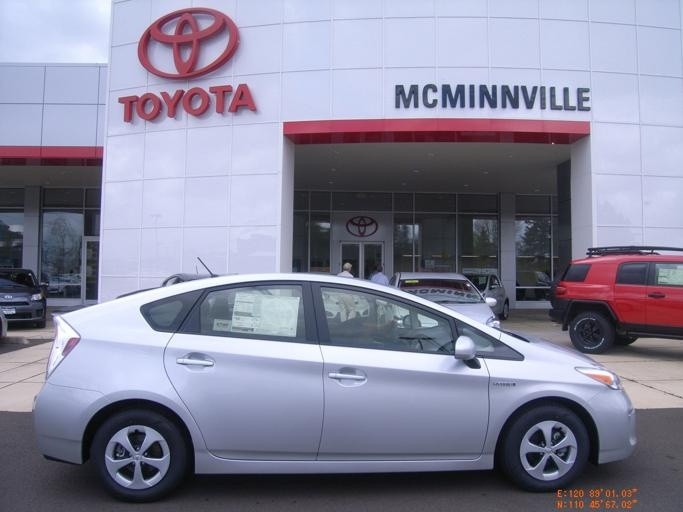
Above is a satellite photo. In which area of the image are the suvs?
[548,243,682,355]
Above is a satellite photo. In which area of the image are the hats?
[343,263,352,269]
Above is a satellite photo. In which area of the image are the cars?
[374,268,501,334]
[31,258,638,503]
[0,268,48,329]
[462,269,510,320]
[516,271,550,300]
[42,276,81,297]
[0,309,7,339]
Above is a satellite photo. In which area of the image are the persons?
[338,262,353,278]
[371,266,389,287]
